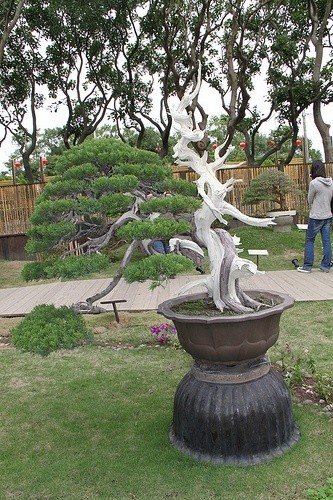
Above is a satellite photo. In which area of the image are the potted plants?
[241,169,304,233]
[158,288,302,468]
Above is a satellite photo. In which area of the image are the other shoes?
[297,266,311,272]
[318,268,328,272]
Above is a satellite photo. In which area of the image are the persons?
[155,240,172,283]
[297,160,332,273]
